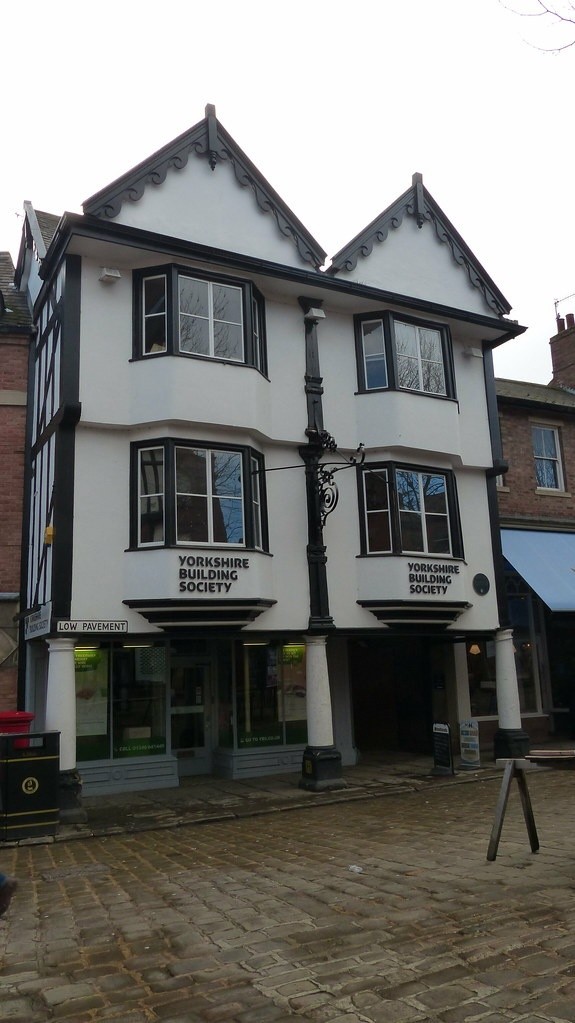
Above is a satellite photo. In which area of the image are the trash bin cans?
[0,729,62,841]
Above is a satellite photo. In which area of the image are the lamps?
[464,347,484,358]
[304,308,326,324]
[100,267,121,283]
